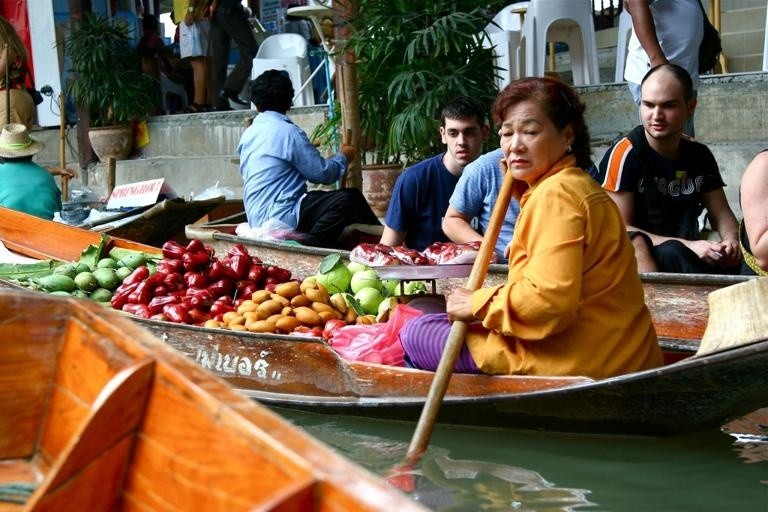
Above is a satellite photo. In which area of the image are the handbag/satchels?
[697,1,722,75]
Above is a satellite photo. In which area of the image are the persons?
[235,68,382,248]
[736,149,768,278]
[0,122,65,222]
[396,76,666,382]
[42,164,81,181]
[596,63,742,274]
[439,144,525,263]
[620,0,706,139]
[0,20,36,138]
[130,1,338,113]
[377,97,490,253]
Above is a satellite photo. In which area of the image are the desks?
[286,3,339,107]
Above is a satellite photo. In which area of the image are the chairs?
[160,70,188,115]
[464,0,601,89]
[248,34,315,112]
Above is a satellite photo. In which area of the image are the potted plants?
[315,0,510,221]
[58,9,157,162]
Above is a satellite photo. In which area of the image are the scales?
[373,264,474,370]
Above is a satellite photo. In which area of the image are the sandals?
[184,102,215,113]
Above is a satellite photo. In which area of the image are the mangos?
[39,254,147,301]
[205,283,376,332]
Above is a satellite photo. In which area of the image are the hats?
[0,123,45,158]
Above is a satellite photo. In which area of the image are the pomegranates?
[303,261,427,314]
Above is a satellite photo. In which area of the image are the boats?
[0,194,224,253]
[1,207,767,435]
[185,210,767,351]
[0,290,430,510]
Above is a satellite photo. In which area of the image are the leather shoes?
[219,88,248,105]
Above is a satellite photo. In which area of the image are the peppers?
[111,240,292,327]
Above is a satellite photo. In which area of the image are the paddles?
[383,169,515,495]
